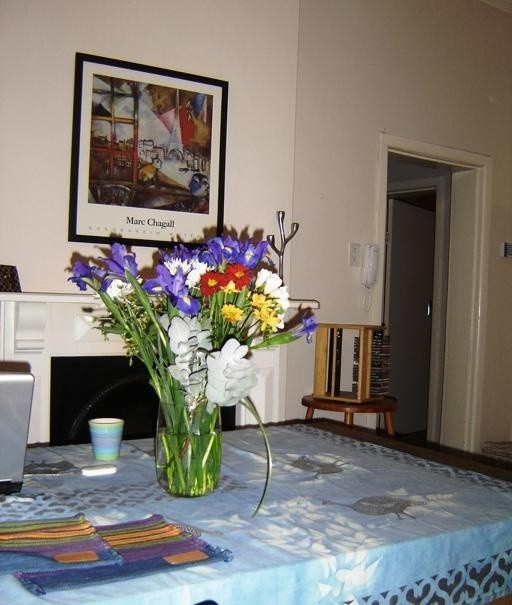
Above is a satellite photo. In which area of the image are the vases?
[156,398,221,497]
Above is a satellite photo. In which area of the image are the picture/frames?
[67,51,229,255]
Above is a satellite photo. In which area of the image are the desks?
[2,416,511,605]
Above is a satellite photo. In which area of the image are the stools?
[302,394,397,438]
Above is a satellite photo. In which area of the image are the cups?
[88,417,126,463]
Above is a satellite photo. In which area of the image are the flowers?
[63,237,319,519]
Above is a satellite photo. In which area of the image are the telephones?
[361,243,378,288]
